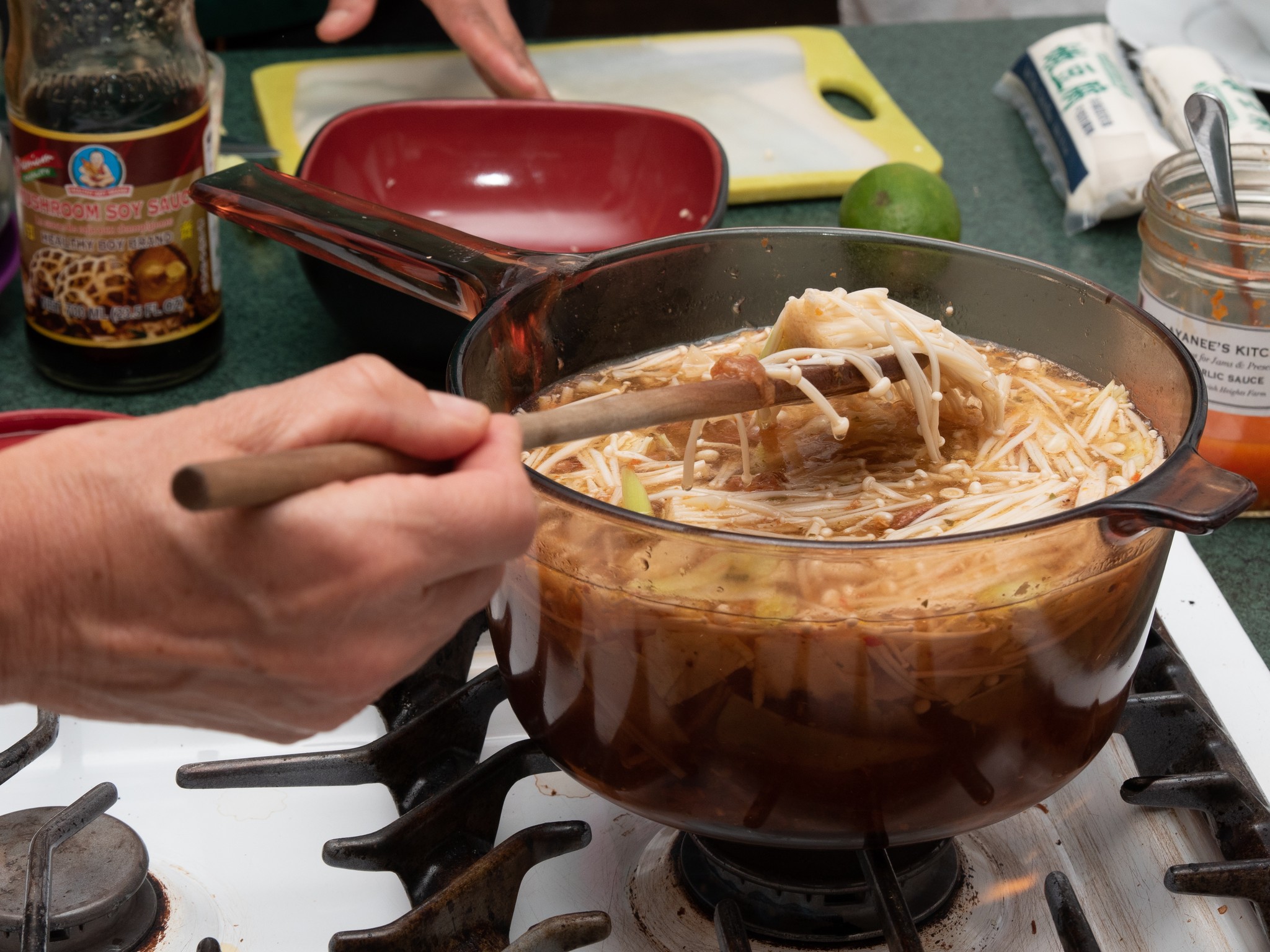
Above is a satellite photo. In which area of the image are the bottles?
[2,0,226,394]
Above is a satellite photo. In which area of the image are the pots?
[188,161,1261,849]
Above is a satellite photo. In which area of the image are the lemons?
[837,162,958,290]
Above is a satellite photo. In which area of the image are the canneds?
[1137,141,1269,521]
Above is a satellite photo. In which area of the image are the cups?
[65,41,226,178]
[1137,143,1270,518]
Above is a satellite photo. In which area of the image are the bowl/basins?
[296,98,730,396]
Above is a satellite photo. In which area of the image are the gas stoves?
[0,524,1270,952]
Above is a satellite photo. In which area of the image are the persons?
[0,0,556,745]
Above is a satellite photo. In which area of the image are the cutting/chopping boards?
[250,26,944,205]
